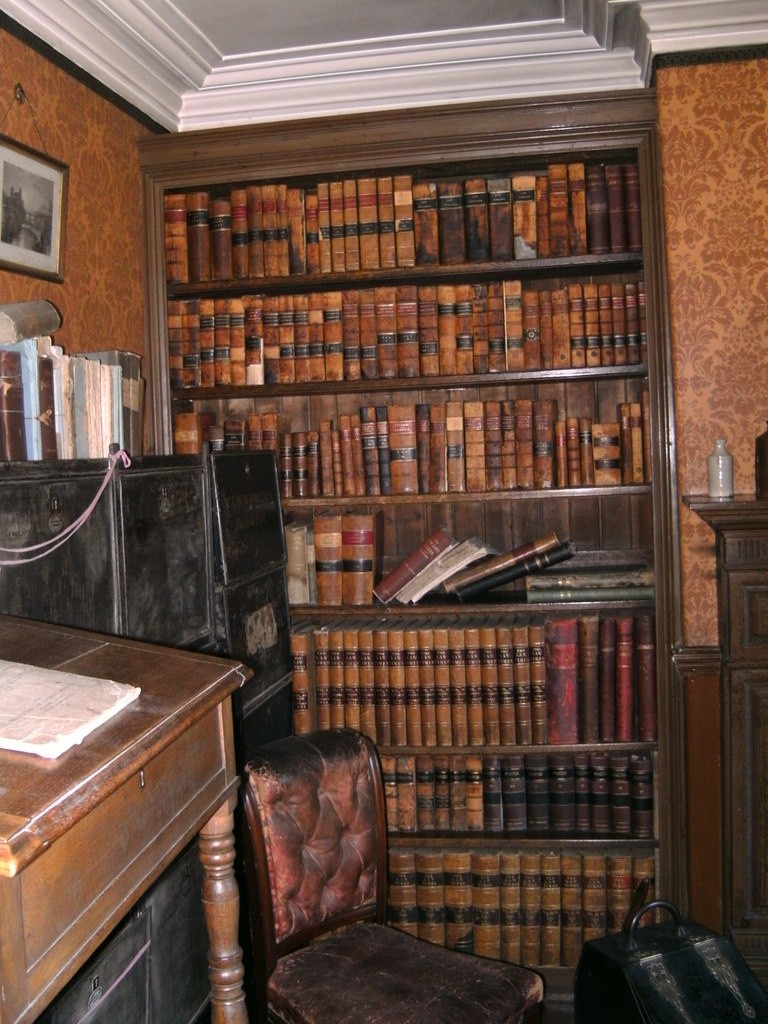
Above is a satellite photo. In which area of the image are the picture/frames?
[0,133,70,284]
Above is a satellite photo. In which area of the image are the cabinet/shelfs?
[133,86,681,1016]
[0,442,294,1024]
[682,493,768,989]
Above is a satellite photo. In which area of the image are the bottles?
[708,438,733,497]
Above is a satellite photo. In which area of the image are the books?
[173,390,651,502]
[387,843,656,970]
[292,611,656,747]
[161,162,653,285]
[167,280,649,389]
[1,334,147,461]
[378,751,654,838]
[285,510,656,605]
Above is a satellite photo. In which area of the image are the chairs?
[232,727,545,1024]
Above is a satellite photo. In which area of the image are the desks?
[0,613,249,1024]
